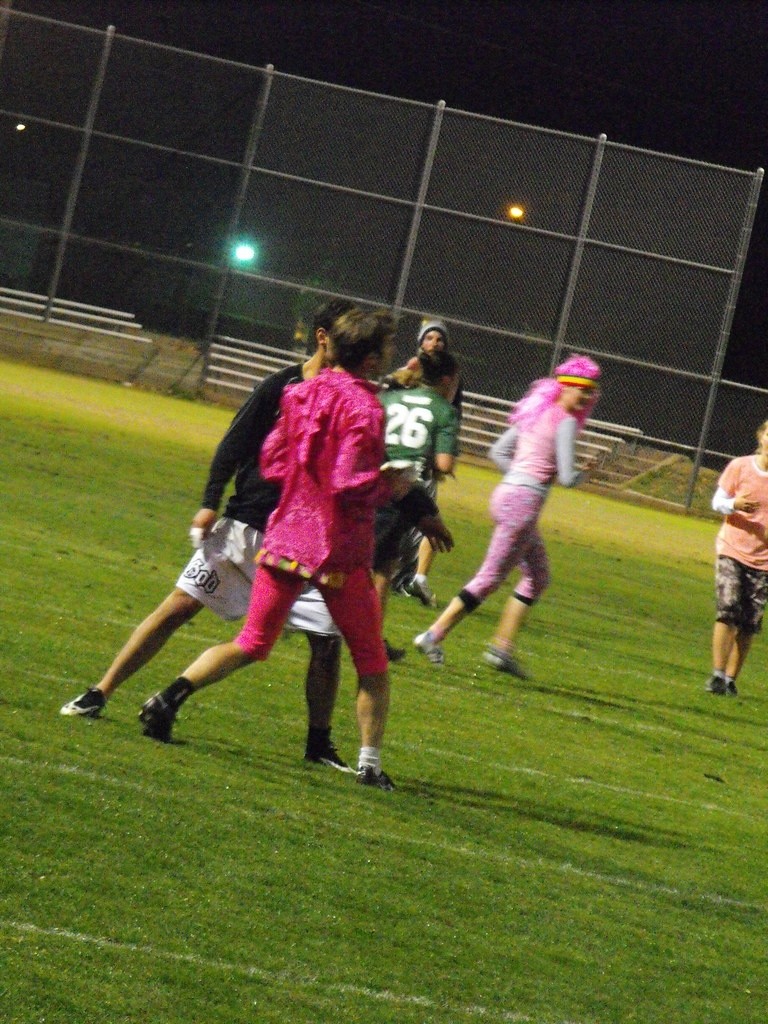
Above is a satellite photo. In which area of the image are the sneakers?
[355,766,394,790]
[60,690,104,718]
[482,647,532,681]
[304,749,355,773]
[414,632,447,665]
[138,694,172,743]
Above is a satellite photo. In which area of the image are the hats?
[417,321,450,350]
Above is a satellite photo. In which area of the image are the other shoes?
[725,678,736,696]
[405,581,437,608]
[703,676,724,694]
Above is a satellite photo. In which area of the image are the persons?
[704,420,768,698]
[138,304,440,792]
[59,301,456,774]
[369,318,603,681]
